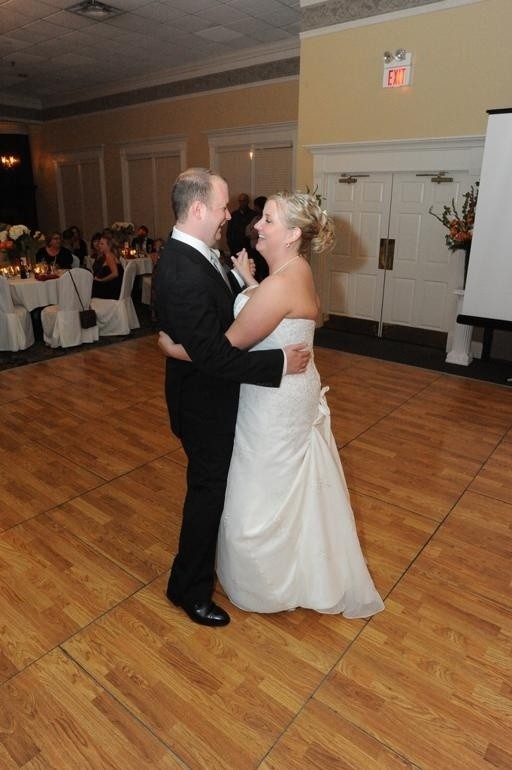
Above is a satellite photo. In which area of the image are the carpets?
[0,298,162,370]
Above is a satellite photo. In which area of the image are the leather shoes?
[165,589,230,627]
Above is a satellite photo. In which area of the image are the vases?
[445,289,473,367]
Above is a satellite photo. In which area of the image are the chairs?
[0,253,152,352]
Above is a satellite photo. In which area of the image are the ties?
[209,248,233,296]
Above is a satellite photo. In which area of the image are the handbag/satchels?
[80,309,97,329]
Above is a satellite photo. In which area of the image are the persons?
[245,196,267,283]
[152,165,312,627]
[35,225,165,305]
[226,193,257,257]
[157,188,384,620]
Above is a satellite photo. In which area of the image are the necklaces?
[272,255,299,274]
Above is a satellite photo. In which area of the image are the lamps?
[82,4,109,18]
[384,49,412,68]
[1,152,17,169]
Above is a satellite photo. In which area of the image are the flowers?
[111,222,135,246]
[429,181,479,290]
[0,223,47,264]
